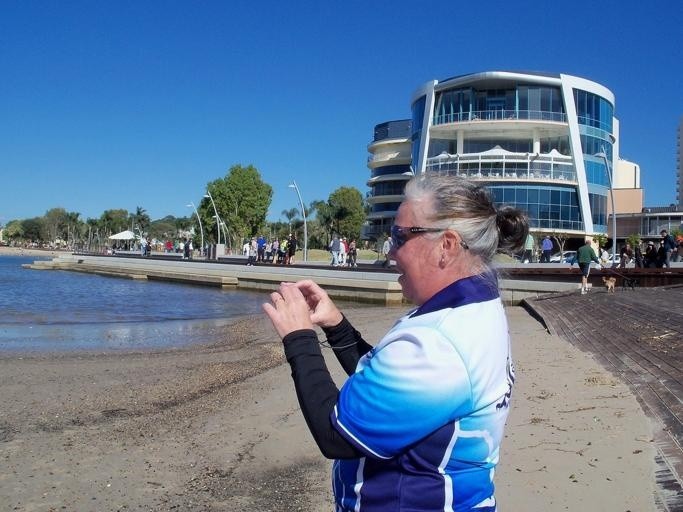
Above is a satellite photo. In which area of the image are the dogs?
[602,277,617,294]
[621,278,636,292]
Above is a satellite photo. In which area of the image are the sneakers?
[585,287,591,291]
[581,291,587,295]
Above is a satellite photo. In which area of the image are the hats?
[343,237,347,239]
[648,241,654,246]
[339,238,343,242]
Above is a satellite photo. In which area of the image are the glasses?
[390,225,469,249]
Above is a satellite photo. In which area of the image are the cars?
[529,249,621,266]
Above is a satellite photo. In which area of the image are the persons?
[600,246,609,263]
[164,237,193,260]
[539,236,553,263]
[112,236,152,256]
[569,240,600,294]
[620,243,632,268]
[635,230,679,268]
[382,235,394,268]
[521,232,534,263]
[262,171,528,512]
[329,234,358,268]
[310,232,327,249]
[243,232,296,266]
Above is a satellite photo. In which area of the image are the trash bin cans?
[208,244,225,260]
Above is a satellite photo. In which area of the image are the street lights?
[593,144,616,266]
[186,201,203,257]
[134,227,142,238]
[203,190,231,250]
[287,178,307,262]
